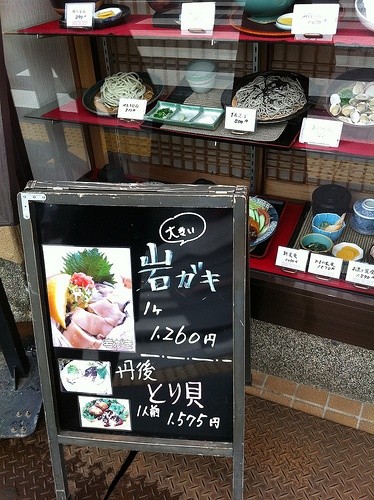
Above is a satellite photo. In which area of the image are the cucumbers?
[248,208,267,233]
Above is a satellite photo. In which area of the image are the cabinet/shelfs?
[0,0,374,349]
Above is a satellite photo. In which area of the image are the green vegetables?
[339,89,355,106]
[304,242,327,252]
[249,200,263,209]
[67,363,108,379]
[62,247,118,286]
[154,108,174,119]
[82,399,128,422]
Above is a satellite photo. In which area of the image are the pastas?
[235,76,307,121]
[99,71,147,108]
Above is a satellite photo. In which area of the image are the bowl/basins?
[49,0,104,18]
[234,0,294,23]
[145,0,195,14]
[325,68,374,136]
[354,0,374,32]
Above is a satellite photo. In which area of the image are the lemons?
[48,274,73,329]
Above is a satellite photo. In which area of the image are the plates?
[249,196,279,247]
[58,4,131,28]
[60,360,109,395]
[93,7,121,19]
[82,72,164,117]
[220,70,317,124]
[229,0,346,37]
[143,100,225,130]
[332,241,364,265]
[152,0,237,28]
[349,215,374,235]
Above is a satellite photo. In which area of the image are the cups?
[183,59,217,94]
[311,213,346,242]
[353,199,374,231]
[312,184,351,219]
[300,233,334,260]
[97,164,123,183]
